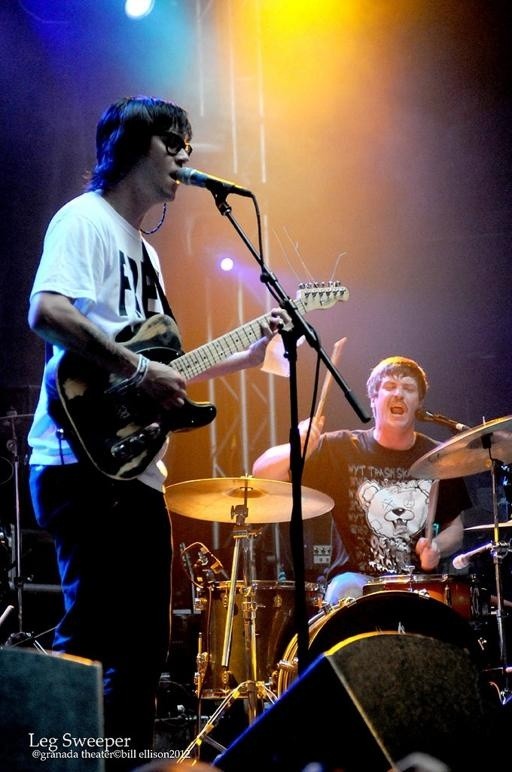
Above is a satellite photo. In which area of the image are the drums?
[200,578,326,700]
[364,571,492,640]
[274,592,480,701]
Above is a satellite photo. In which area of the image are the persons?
[22,95,290,770]
[252,355,512,612]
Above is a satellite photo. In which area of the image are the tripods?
[0,411,48,655]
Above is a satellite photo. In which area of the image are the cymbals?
[164,478,334,526]
[1,414,35,427]
[410,413,512,481]
[464,517,512,530]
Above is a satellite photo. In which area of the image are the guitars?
[44,280,350,482]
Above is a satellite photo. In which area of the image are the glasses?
[153,130,193,157]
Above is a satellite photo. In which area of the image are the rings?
[276,320,286,333]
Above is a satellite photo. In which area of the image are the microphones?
[452,543,492,569]
[414,408,470,432]
[175,167,251,198]
